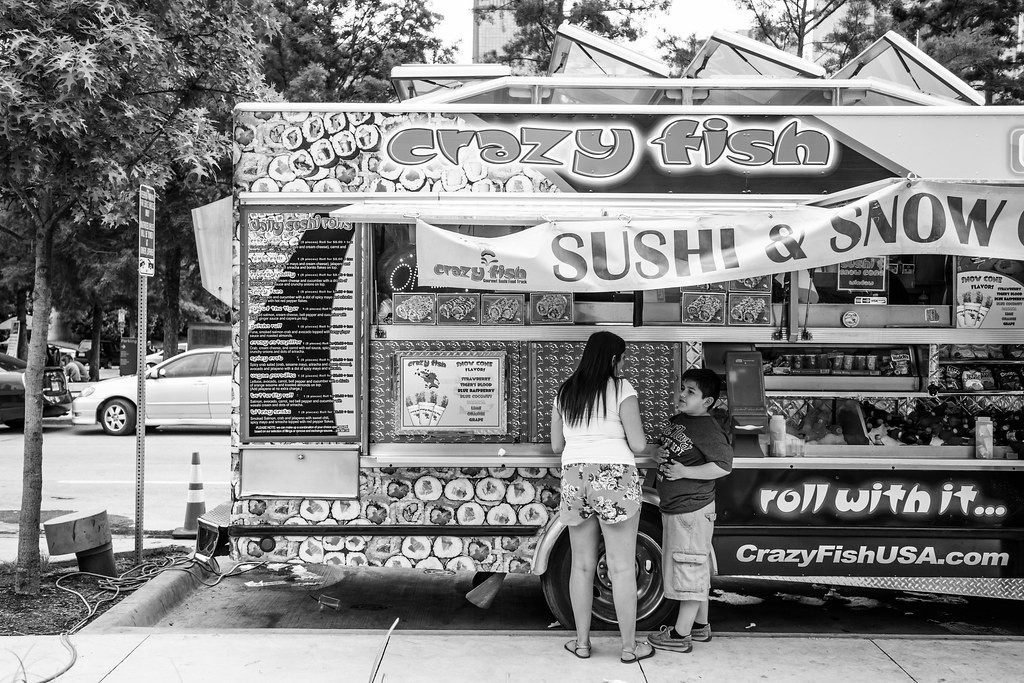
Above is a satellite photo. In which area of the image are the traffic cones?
[172,451,206,540]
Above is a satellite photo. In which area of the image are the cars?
[70,346,233,435]
[0,353,72,429]
[146,345,186,370]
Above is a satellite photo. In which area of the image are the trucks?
[190,23,1024,632]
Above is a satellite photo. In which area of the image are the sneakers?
[691,623,712,642]
[646,625,693,653]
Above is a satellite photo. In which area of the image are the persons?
[645,368,733,654]
[550,330,656,664]
[60,355,92,380]
[772,270,818,304]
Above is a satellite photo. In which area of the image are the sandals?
[565,640,591,658]
[621,641,655,664]
